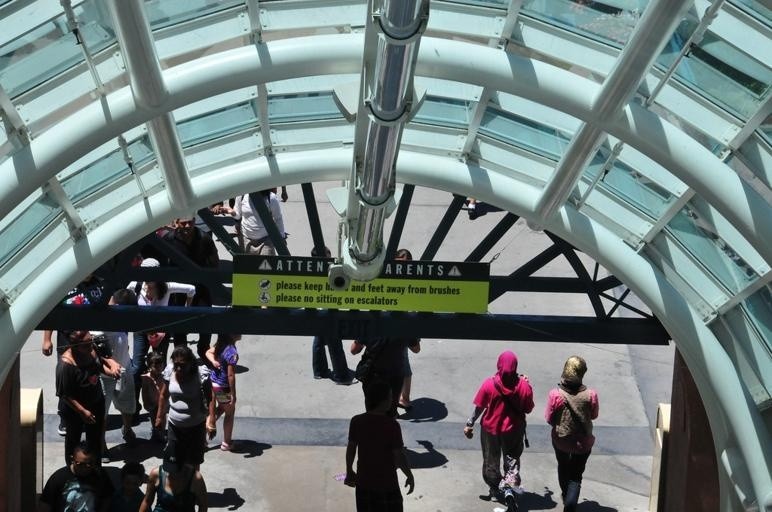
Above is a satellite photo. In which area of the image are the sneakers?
[504,489,518,512]
[490,488,500,502]
[221,439,242,451]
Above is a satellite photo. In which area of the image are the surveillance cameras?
[327,265,352,289]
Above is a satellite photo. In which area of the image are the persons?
[466,198,477,216]
[210,186,292,256]
[309,244,359,387]
[344,377,417,512]
[395,249,413,263]
[350,334,418,420]
[462,350,533,510]
[38,217,241,512]
[544,354,599,510]
